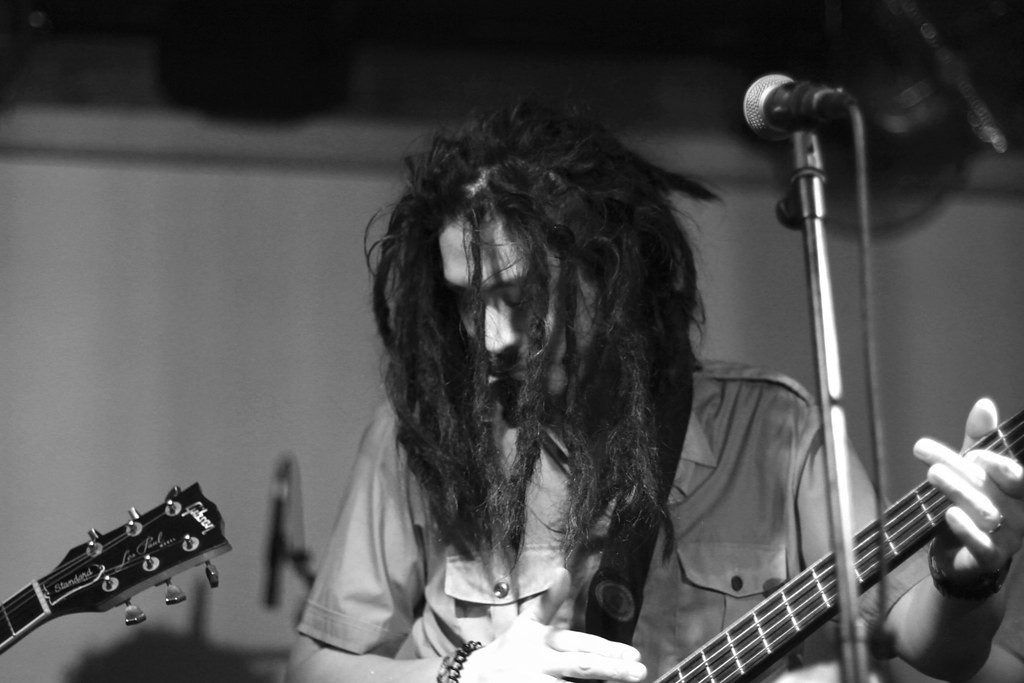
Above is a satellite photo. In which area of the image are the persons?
[285,93,1024,683]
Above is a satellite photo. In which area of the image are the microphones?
[744,73,854,142]
[261,472,289,615]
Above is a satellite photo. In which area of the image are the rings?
[988,516,1005,534]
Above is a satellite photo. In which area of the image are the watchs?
[435,640,482,683]
[928,533,1012,601]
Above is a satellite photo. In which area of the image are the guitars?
[0,479,234,660]
[656,412,1022,683]
